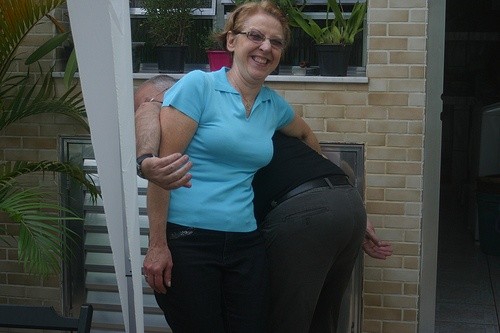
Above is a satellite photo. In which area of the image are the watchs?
[136,153,160,179]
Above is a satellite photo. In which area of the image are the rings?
[144,274,148,278]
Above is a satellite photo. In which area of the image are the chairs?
[465,102,500,246]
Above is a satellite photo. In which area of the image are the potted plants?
[141,0,195,74]
[198,27,232,71]
[60,13,78,72]
[294,0,366,77]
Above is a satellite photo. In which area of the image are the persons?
[134,74,394,333]
[144,0,322,333]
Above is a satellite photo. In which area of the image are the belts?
[257,177,350,219]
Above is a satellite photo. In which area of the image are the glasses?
[144,85,172,102]
[235,30,287,49]
